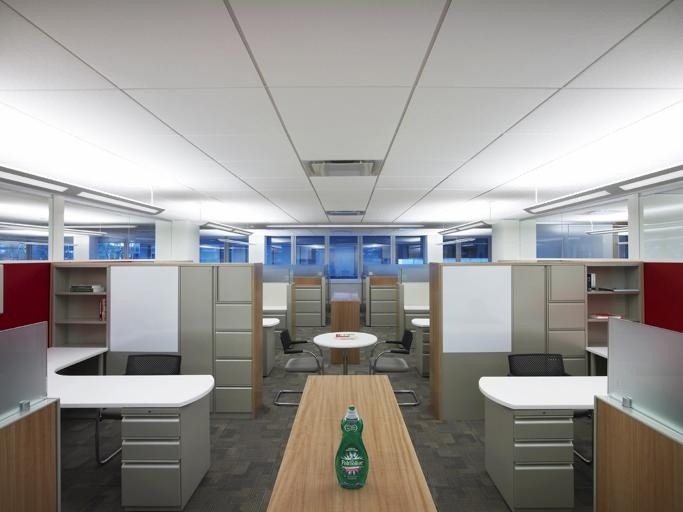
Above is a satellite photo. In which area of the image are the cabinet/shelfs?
[586,259,644,346]
[52,262,110,349]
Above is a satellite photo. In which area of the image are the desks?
[313,332,377,375]
[330,292,362,332]
[263,306,286,378]
[47,347,215,512]
[404,304,429,377]
[479,347,607,512]
[266,376,436,512]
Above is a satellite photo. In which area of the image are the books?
[70,284,100,288]
[69,287,104,292]
[101,298,106,321]
[598,287,640,292]
[99,301,101,320]
[591,273,595,291]
[335,333,355,339]
[586,273,591,291]
[592,312,623,320]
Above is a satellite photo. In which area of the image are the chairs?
[97,355,180,467]
[508,354,593,463]
[273,330,324,406]
[369,328,420,406]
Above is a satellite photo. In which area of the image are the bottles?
[334,403,369,490]
